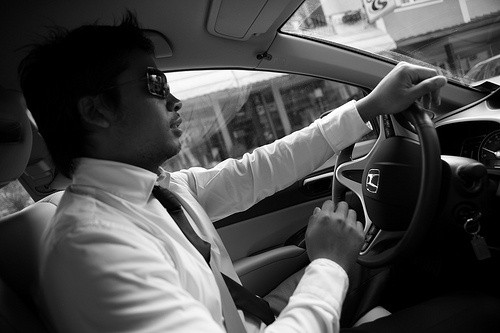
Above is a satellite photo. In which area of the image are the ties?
[152,184,276,326]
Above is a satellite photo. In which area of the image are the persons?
[19,10,446,333]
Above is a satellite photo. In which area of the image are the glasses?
[90,67,169,99]
